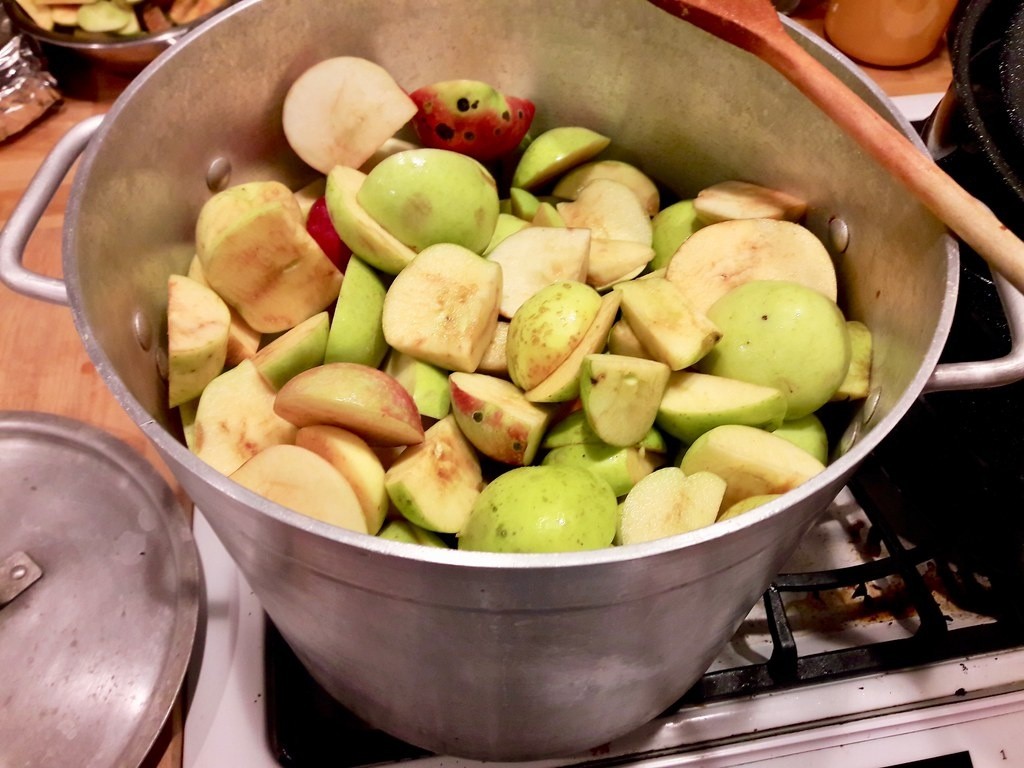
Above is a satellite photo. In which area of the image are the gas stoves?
[181,83,1022,768]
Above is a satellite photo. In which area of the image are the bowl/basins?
[9,0,236,74]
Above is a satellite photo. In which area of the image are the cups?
[824,0,954,68]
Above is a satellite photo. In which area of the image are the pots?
[3,0,1022,761]
